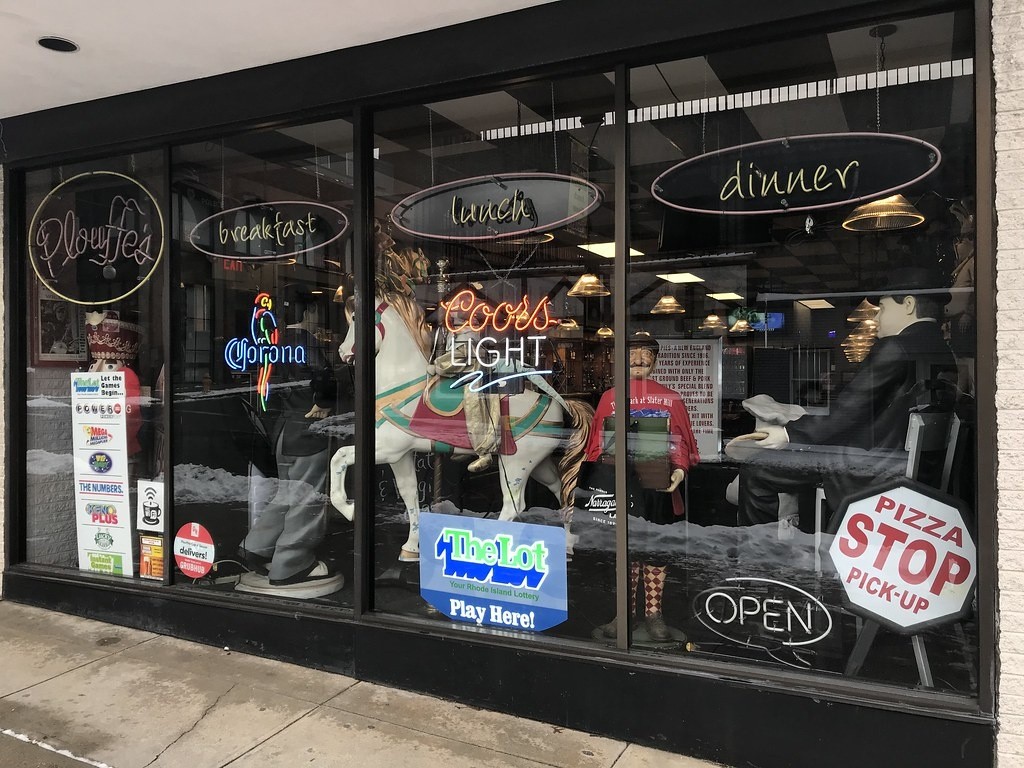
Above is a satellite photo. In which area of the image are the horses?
[329,272,596,564]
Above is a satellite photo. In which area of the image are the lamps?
[729,264,755,333]
[505,281,531,324]
[333,283,344,304]
[840,337,874,347]
[593,321,614,339]
[698,268,727,328]
[566,150,611,296]
[841,25,925,232]
[649,273,686,314]
[849,320,881,336]
[844,346,870,363]
[846,298,880,322]
[556,291,581,331]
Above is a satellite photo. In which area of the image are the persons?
[85,319,145,572]
[584,334,700,643]
[738,267,958,535]
[236,284,337,586]
